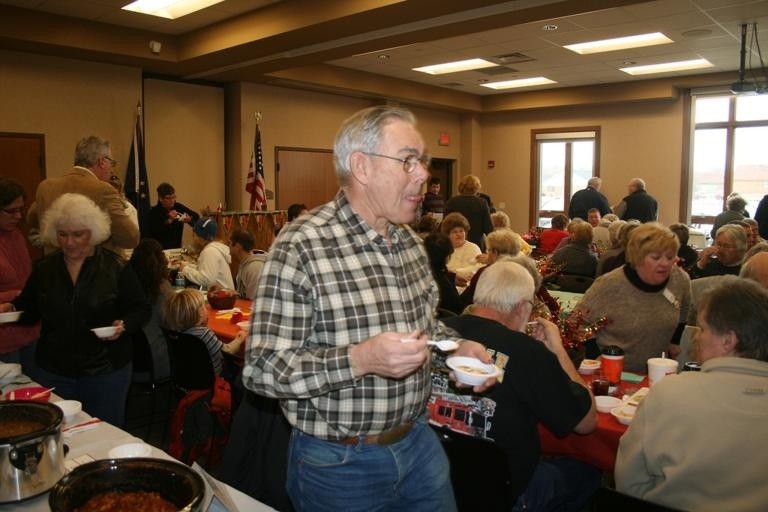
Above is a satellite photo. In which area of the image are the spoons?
[402,336,458,354]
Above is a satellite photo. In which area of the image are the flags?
[126,109,149,209]
[246,123,271,212]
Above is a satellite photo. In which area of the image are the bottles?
[591,378,609,396]
[175,270,185,289]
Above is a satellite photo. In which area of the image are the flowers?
[521,224,543,247]
[532,257,562,278]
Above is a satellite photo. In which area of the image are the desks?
[158,287,654,472]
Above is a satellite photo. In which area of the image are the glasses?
[163,195,178,200]
[485,248,494,255]
[106,156,117,167]
[1,206,29,214]
[528,300,537,315]
[361,150,432,174]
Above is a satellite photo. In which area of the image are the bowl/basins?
[593,396,623,415]
[235,320,251,333]
[206,290,237,311]
[446,354,500,387]
[48,457,205,511]
[0,311,24,323]
[89,326,119,337]
[0,363,83,418]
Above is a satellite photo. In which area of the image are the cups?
[599,344,625,393]
[682,361,702,372]
[645,358,678,390]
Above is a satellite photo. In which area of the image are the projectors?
[730,82,764,94]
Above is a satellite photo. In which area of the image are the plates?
[614,387,650,424]
[576,359,601,375]
[107,443,151,459]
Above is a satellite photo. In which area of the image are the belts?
[341,421,415,447]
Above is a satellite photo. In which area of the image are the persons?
[1,194,151,430]
[444,175,495,241]
[242,102,509,512]
[152,183,200,249]
[569,177,613,222]
[125,192,768,512]
[610,178,658,223]
[612,274,767,511]
[480,191,496,215]
[422,178,445,213]
[25,136,141,262]
[0,179,40,370]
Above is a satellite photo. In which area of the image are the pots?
[0,398,70,504]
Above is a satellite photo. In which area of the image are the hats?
[193,216,217,242]
